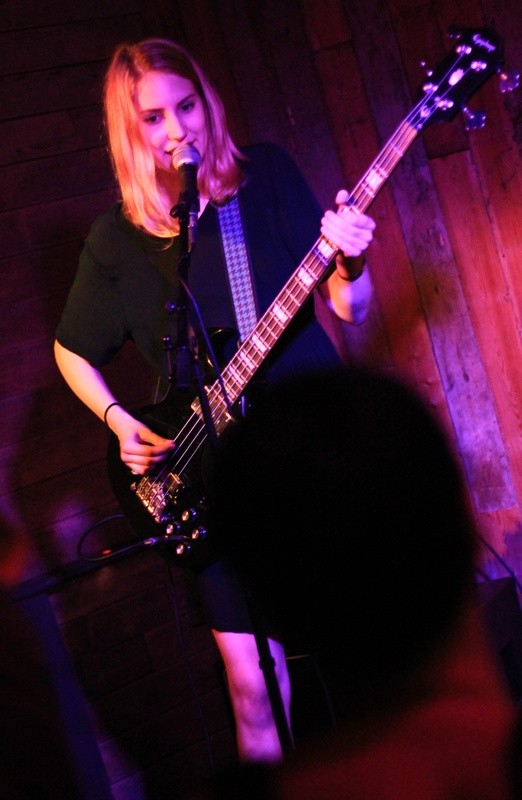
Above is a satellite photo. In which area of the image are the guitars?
[105,22,522,568]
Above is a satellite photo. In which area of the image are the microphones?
[172,144,204,244]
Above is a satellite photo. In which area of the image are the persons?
[54,40,378,759]
[195,364,521,800]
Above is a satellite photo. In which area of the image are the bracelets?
[337,256,367,282]
[104,402,122,427]
